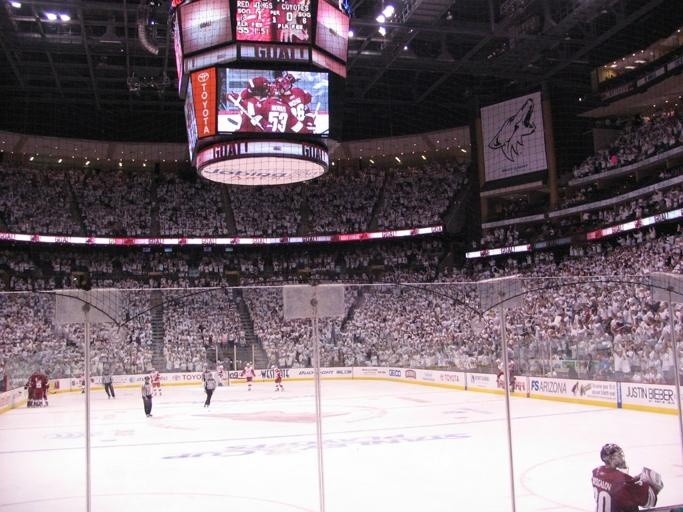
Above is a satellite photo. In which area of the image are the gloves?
[249,114,266,131]
[304,116,316,131]
[224,91,240,103]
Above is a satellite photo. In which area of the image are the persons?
[592,442,658,512]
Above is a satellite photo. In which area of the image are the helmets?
[274,78,290,93]
[246,76,270,97]
[600,443,621,464]
